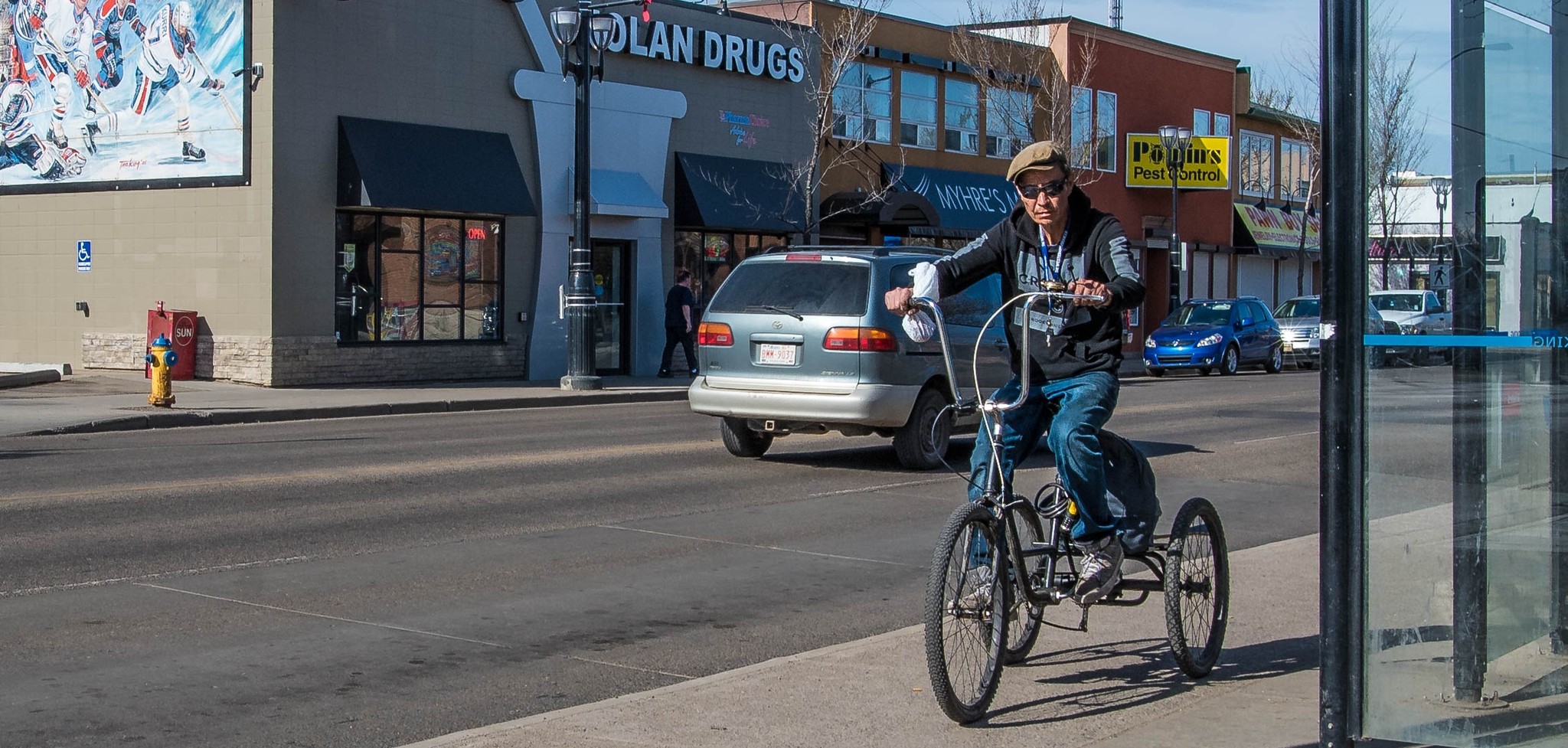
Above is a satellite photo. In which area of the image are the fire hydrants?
[144,332,178,408]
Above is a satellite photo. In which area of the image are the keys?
[1045,328,1054,347]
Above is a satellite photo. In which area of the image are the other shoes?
[689,369,699,378]
[658,370,674,378]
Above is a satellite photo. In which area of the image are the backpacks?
[1098,429,1162,553]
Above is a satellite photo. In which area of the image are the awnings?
[1233,203,1320,262]
[674,152,819,233]
[880,163,1021,238]
[337,117,539,216]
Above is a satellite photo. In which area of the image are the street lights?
[1157,124,1194,315]
[1430,176,1452,314]
[547,0,617,392]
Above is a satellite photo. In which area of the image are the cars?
[1140,295,1284,377]
[1271,294,1389,372]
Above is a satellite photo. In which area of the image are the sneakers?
[1074,535,1124,603]
[947,567,1018,620]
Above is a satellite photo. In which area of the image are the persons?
[885,141,1145,620]
[657,271,699,378]
[592,275,606,332]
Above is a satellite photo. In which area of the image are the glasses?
[1016,176,1068,199]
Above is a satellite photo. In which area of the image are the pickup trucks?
[1369,289,1453,368]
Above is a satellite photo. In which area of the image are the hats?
[1006,141,1063,185]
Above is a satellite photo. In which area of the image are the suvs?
[687,241,1016,473]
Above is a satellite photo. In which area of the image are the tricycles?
[901,275,1232,725]
